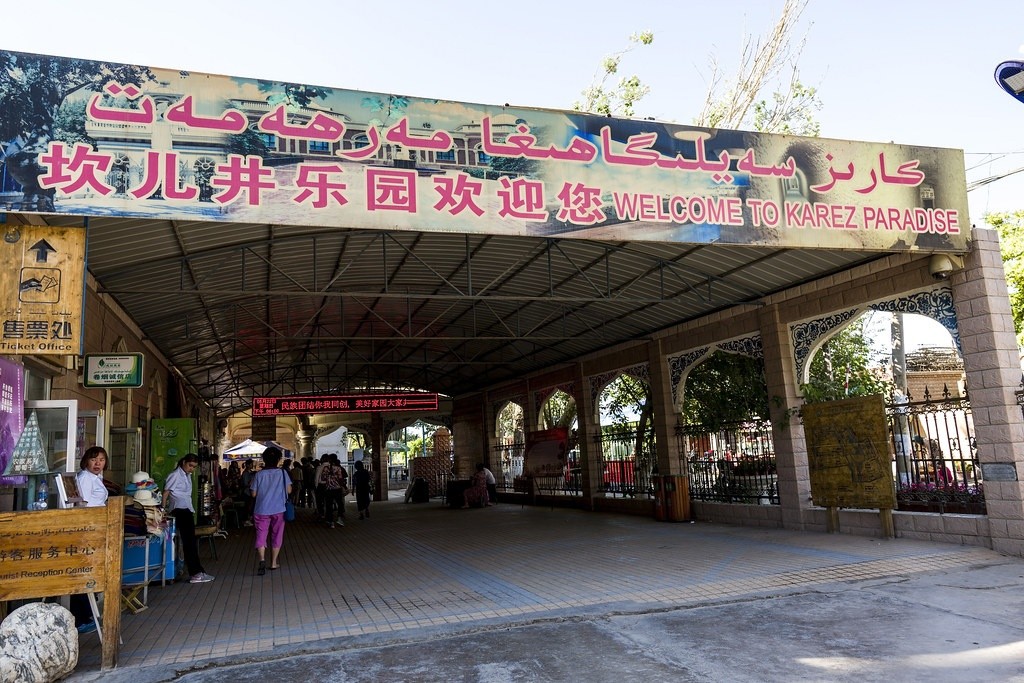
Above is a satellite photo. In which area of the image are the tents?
[223,436,294,468]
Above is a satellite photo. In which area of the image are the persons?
[482,467,499,503]
[70,446,109,637]
[462,463,490,509]
[249,447,293,575]
[221,453,349,528]
[162,453,215,583]
[936,460,953,484]
[352,460,372,519]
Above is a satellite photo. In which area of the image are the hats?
[123,471,166,536]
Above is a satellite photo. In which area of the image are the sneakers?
[78,617,102,634]
[189,572,215,584]
[243,521,254,527]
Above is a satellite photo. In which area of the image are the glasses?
[202,486,215,511]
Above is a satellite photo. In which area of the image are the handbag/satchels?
[340,483,349,497]
[285,498,295,520]
[326,474,341,490]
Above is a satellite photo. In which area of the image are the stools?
[223,508,240,529]
[121,584,148,614]
[195,524,217,560]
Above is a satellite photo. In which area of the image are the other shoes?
[487,502,493,506]
[336,520,345,527]
[271,564,280,569]
[366,511,370,518]
[330,522,336,528]
[358,515,365,520]
[257,561,265,576]
[461,505,471,508]
